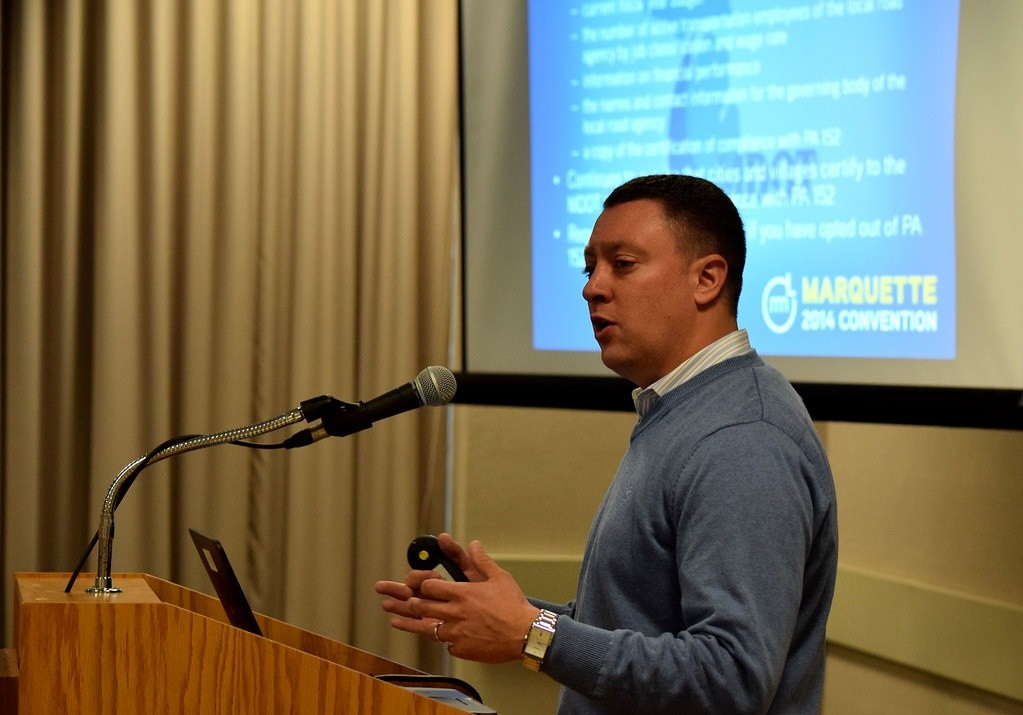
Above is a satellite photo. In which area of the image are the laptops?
[187,526,497,715]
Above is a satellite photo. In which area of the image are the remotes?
[407,534,470,585]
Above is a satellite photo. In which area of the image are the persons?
[376,174,838,715]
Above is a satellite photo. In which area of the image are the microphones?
[284,366,456,449]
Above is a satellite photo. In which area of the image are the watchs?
[521,608,559,673]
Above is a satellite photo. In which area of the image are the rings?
[434,622,446,643]
